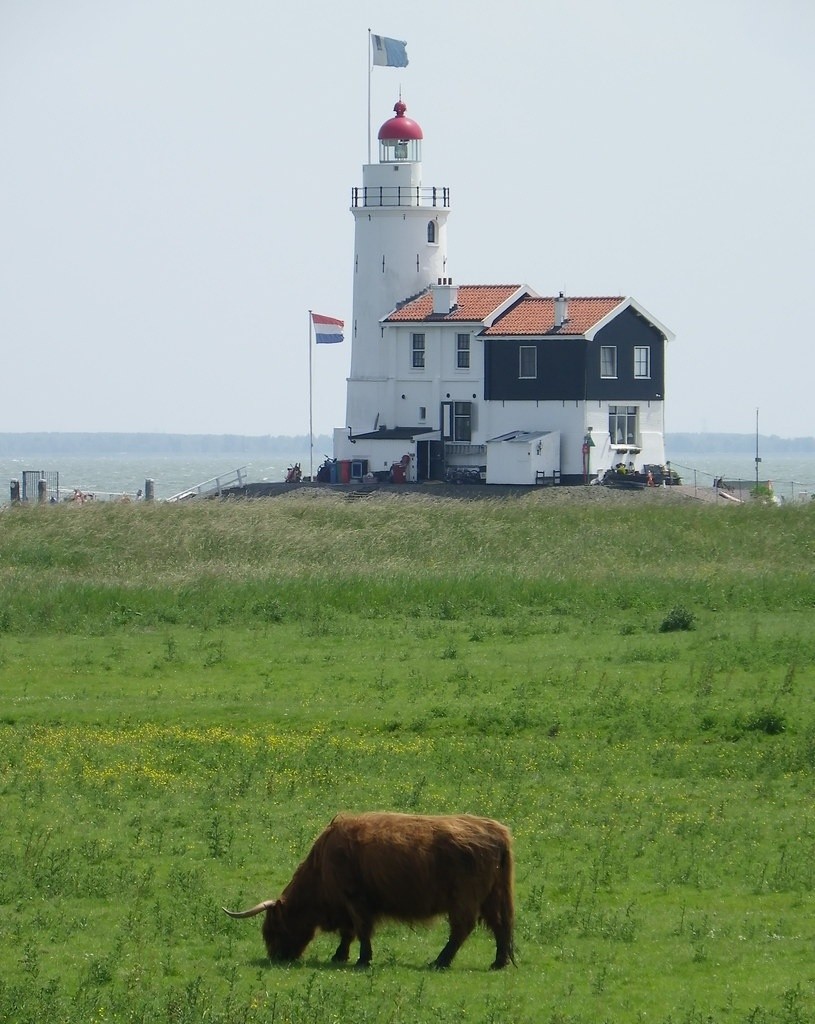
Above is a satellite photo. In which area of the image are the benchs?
[644,462,682,485]
[535,470,562,485]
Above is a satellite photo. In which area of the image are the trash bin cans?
[392,455,409,484]
[329,459,351,484]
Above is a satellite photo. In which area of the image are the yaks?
[221,811,523,971]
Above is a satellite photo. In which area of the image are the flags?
[371,35,409,67]
[312,314,345,344]
